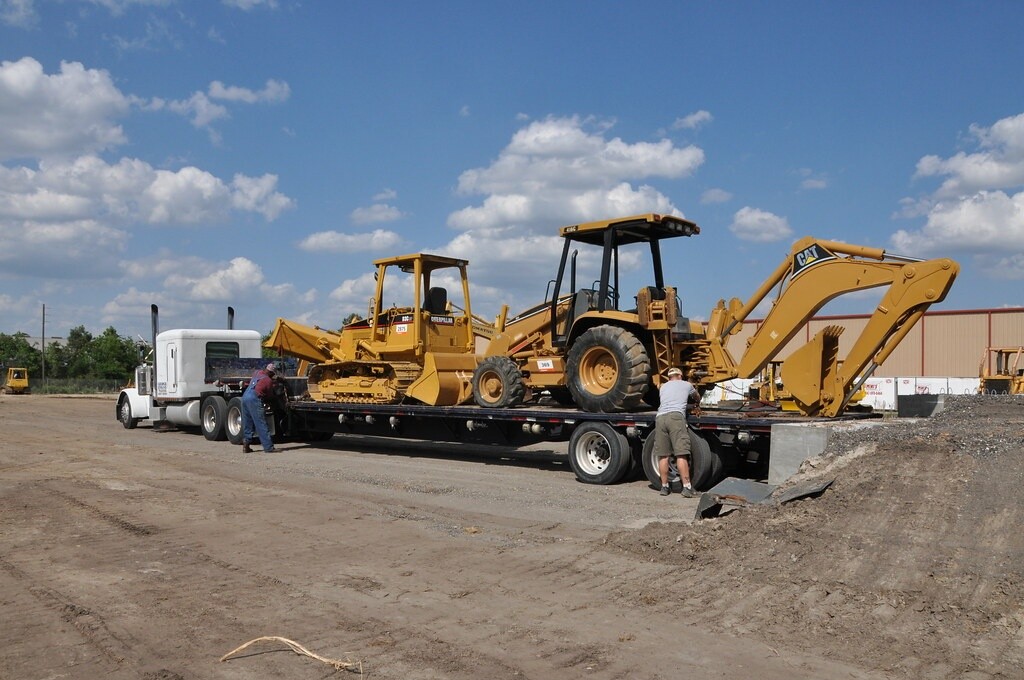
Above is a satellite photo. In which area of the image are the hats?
[267,363,277,374]
[668,367,684,376]
[238,381,243,389]
[14,373,17,377]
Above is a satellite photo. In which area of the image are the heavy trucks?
[116,303,884,495]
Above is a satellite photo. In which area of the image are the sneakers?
[660,485,672,496]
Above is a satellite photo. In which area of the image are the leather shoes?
[681,486,702,497]
[243,443,253,453]
[265,446,281,452]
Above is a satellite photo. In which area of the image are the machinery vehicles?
[978,346,1024,396]
[404,213,960,419]
[747,360,872,413]
[263,254,470,406]
[0,368,32,395]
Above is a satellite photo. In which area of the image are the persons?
[241,364,282,453]
[655,368,702,498]
[14,371,22,379]
